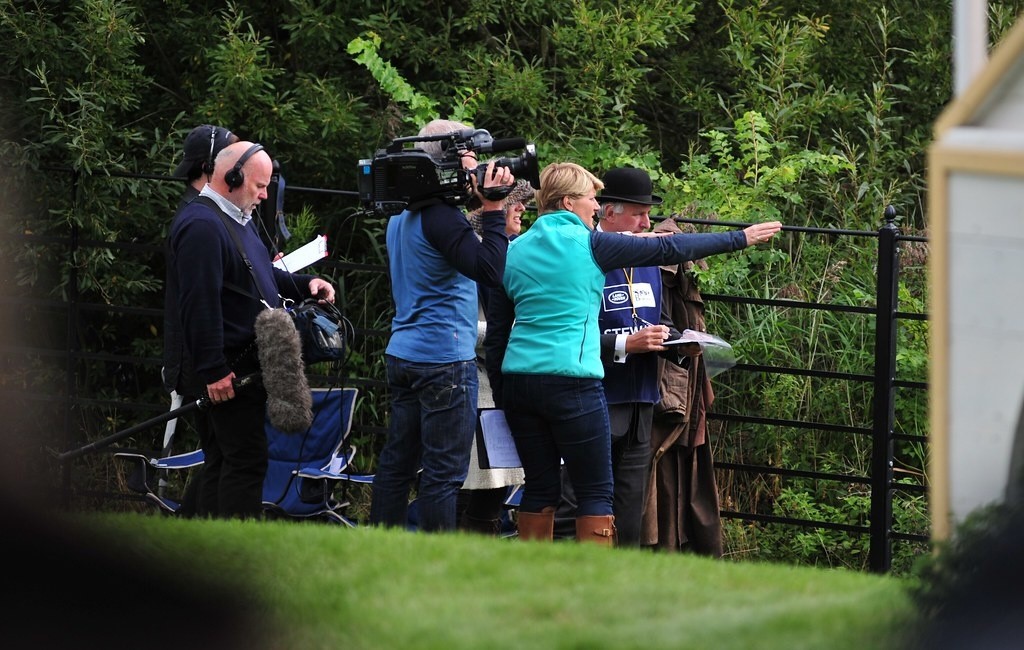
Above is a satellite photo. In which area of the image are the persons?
[468,162,783,561]
[368,118,516,534]
[166,126,337,522]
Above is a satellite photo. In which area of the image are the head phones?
[203,125,217,175]
[224,143,263,194]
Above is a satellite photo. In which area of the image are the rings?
[501,179,508,184]
[757,237,760,242]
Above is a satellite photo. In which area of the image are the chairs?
[114,387,375,528]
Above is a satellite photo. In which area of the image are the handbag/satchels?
[290,307,346,365]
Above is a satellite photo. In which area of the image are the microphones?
[470,138,525,155]
[254,307,314,435]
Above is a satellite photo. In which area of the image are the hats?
[593,168,663,205]
[173,125,233,177]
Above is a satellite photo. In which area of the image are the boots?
[516,507,553,542]
[575,513,617,547]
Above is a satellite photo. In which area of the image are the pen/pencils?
[633,316,673,336]
